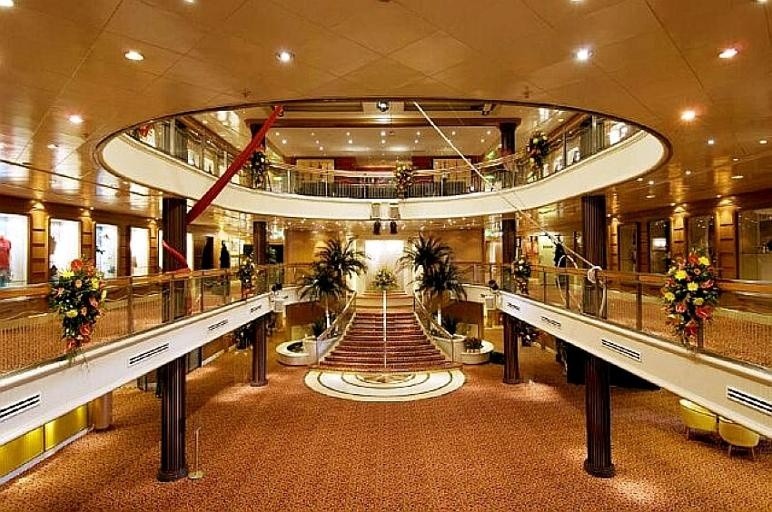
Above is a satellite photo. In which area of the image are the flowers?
[44,256,110,369]
[660,247,722,350]
[233,255,260,295]
[511,256,530,295]
[527,129,552,169]
[250,150,272,187]
[389,164,415,199]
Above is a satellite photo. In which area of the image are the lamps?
[373,219,381,235]
[390,221,397,234]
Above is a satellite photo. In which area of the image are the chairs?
[679,399,717,440]
[718,416,760,461]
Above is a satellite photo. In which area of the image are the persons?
[0,233,12,286]
[155,368,162,398]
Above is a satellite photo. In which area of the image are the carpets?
[304,370,466,402]
